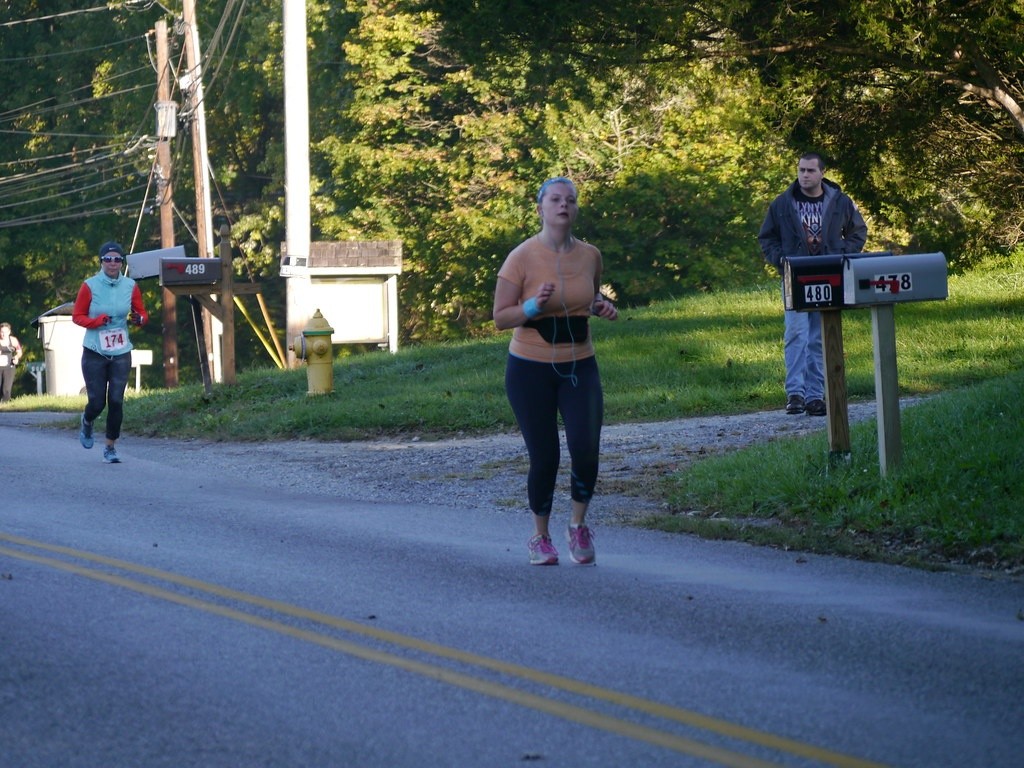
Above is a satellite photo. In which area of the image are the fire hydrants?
[290,308,338,396]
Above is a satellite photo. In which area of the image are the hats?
[98,242,123,263]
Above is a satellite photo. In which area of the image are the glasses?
[100,256,124,263]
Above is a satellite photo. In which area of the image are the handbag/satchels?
[523,315,591,344]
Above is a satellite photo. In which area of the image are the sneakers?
[103,445,121,464]
[563,523,595,566]
[526,534,558,566]
[80,412,93,448]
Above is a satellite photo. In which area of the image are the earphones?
[540,211,545,218]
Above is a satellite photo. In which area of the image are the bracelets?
[523,296,541,319]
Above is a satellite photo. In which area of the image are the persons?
[72,240,148,465]
[0,322,23,404]
[757,151,869,416]
[492,178,619,567]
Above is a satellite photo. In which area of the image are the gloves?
[103,315,112,327]
[128,309,143,326]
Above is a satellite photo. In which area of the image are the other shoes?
[786,395,805,414]
[805,398,827,415]
